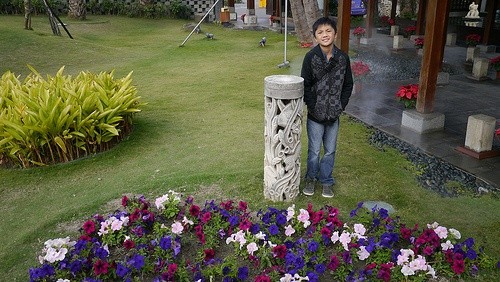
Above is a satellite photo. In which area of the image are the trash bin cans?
[221,8,230,22]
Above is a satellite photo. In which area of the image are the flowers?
[352,26,365,39]
[381,15,395,30]
[463,33,480,47]
[487,55,500,72]
[404,25,417,35]
[270,16,280,23]
[350,61,369,82]
[413,37,424,49]
[395,82,419,108]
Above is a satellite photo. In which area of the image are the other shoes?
[321,185,334,198]
[302,176,317,196]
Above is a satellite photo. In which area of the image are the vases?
[353,80,362,96]
[496,71,500,79]
[273,23,279,27]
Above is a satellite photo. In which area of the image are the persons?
[302,17,353,197]
